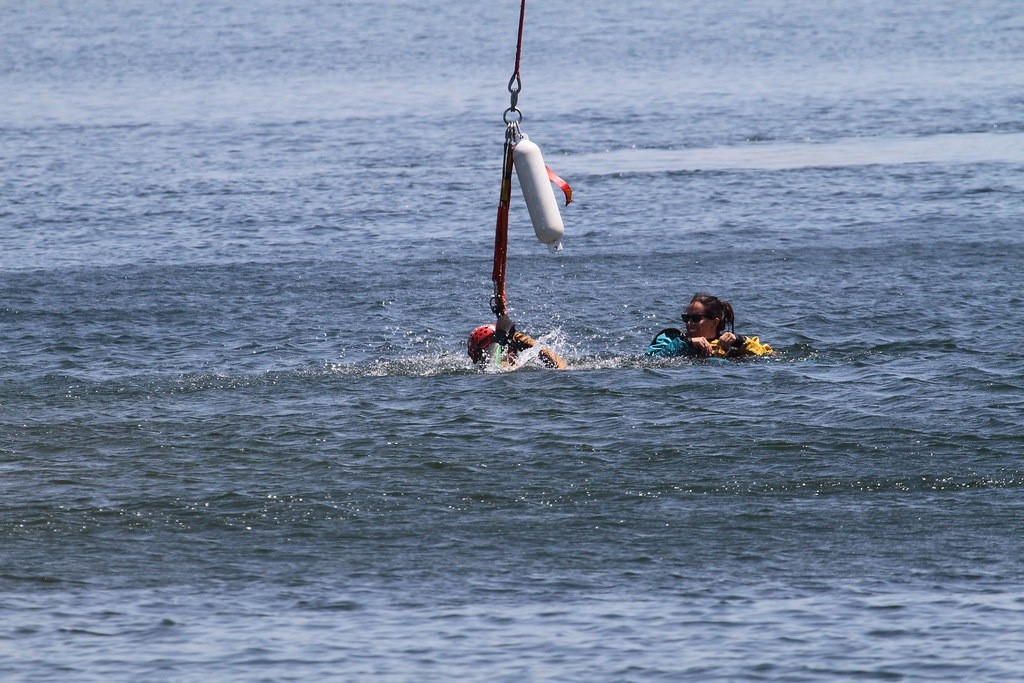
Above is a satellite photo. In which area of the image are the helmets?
[466,323,497,364]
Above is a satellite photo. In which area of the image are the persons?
[645,294,774,367]
[468,323,565,369]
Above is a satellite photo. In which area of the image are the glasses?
[681,313,716,323]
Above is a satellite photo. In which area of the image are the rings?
[702,341,706,344]
